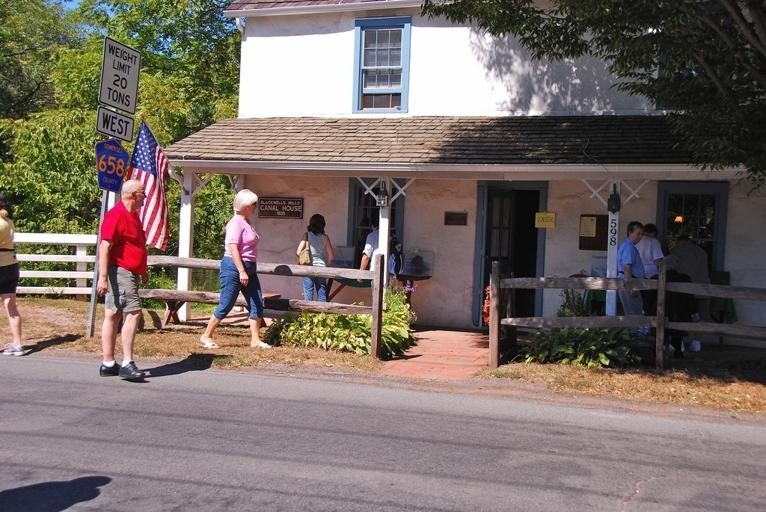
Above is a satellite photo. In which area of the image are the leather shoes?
[100,361,145,383]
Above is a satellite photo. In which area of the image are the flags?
[126,120,170,253]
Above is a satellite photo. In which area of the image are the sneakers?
[654,341,701,359]
[4,345,25,356]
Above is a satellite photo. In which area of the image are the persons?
[296,214,335,302]
[0,193,24,356]
[200,189,272,350]
[357,216,403,283]
[97,179,149,379]
[617,221,708,358]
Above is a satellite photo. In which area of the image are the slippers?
[199,343,220,348]
[255,343,271,348]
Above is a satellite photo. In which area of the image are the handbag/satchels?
[299,232,311,264]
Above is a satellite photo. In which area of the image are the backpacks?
[389,232,404,275]
